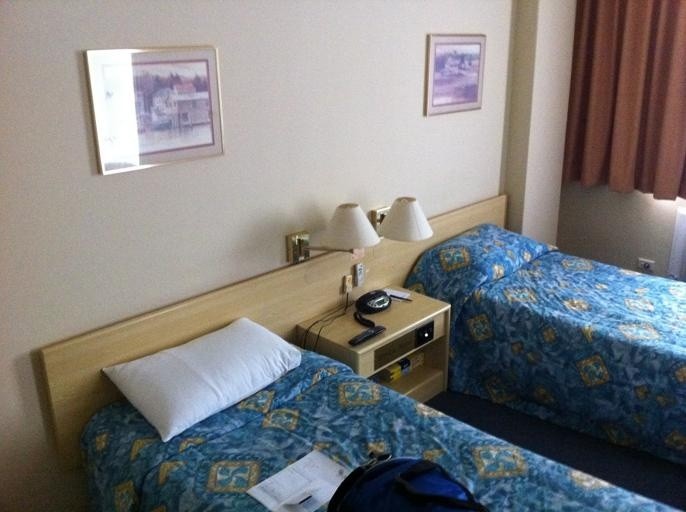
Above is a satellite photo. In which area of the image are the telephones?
[355,290,392,314]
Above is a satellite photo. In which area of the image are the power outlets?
[635,255,658,274]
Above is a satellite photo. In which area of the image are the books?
[378,349,425,384]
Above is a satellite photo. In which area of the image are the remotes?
[348,326,386,345]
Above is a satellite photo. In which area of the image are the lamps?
[277,197,383,267]
[367,193,439,249]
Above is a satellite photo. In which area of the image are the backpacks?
[326,454,490,512]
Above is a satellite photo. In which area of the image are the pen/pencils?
[390,295,413,301]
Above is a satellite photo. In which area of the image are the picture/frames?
[81,43,229,181]
[416,31,490,120]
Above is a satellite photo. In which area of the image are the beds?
[76,338,685,512]
[398,221,685,468]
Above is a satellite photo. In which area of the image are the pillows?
[97,313,302,442]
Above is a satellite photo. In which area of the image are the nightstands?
[291,280,453,411]
